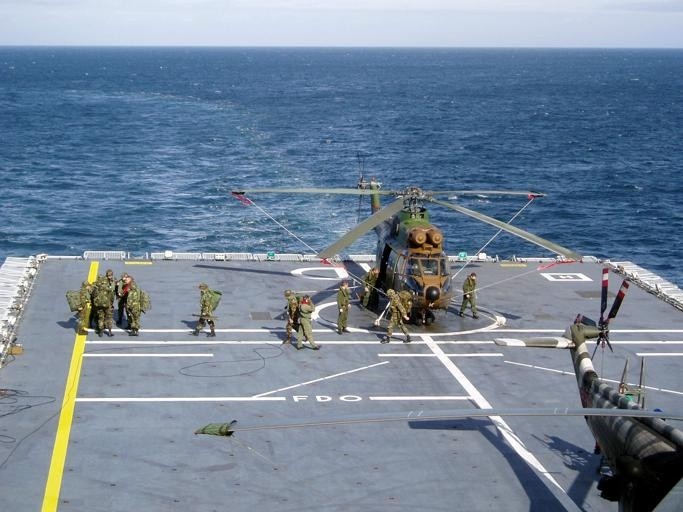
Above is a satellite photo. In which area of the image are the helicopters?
[192,267,683,511]
[232,178,585,324]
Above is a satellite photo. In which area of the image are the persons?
[337,279,352,335]
[77,269,143,336]
[355,267,381,312]
[283,289,309,344]
[296,294,323,350]
[189,282,217,337]
[457,272,481,320]
[379,288,412,344]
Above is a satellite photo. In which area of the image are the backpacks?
[93,275,110,309]
[397,290,412,314]
[210,290,223,312]
[64,288,81,313]
[139,288,151,313]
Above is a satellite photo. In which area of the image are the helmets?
[282,289,291,298]
[79,280,91,288]
[197,282,207,291]
[384,288,395,297]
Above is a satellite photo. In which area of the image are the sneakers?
[460,311,480,318]
[380,334,411,345]
[190,329,216,338]
[77,320,138,337]
[282,337,321,351]
[337,326,350,335]
